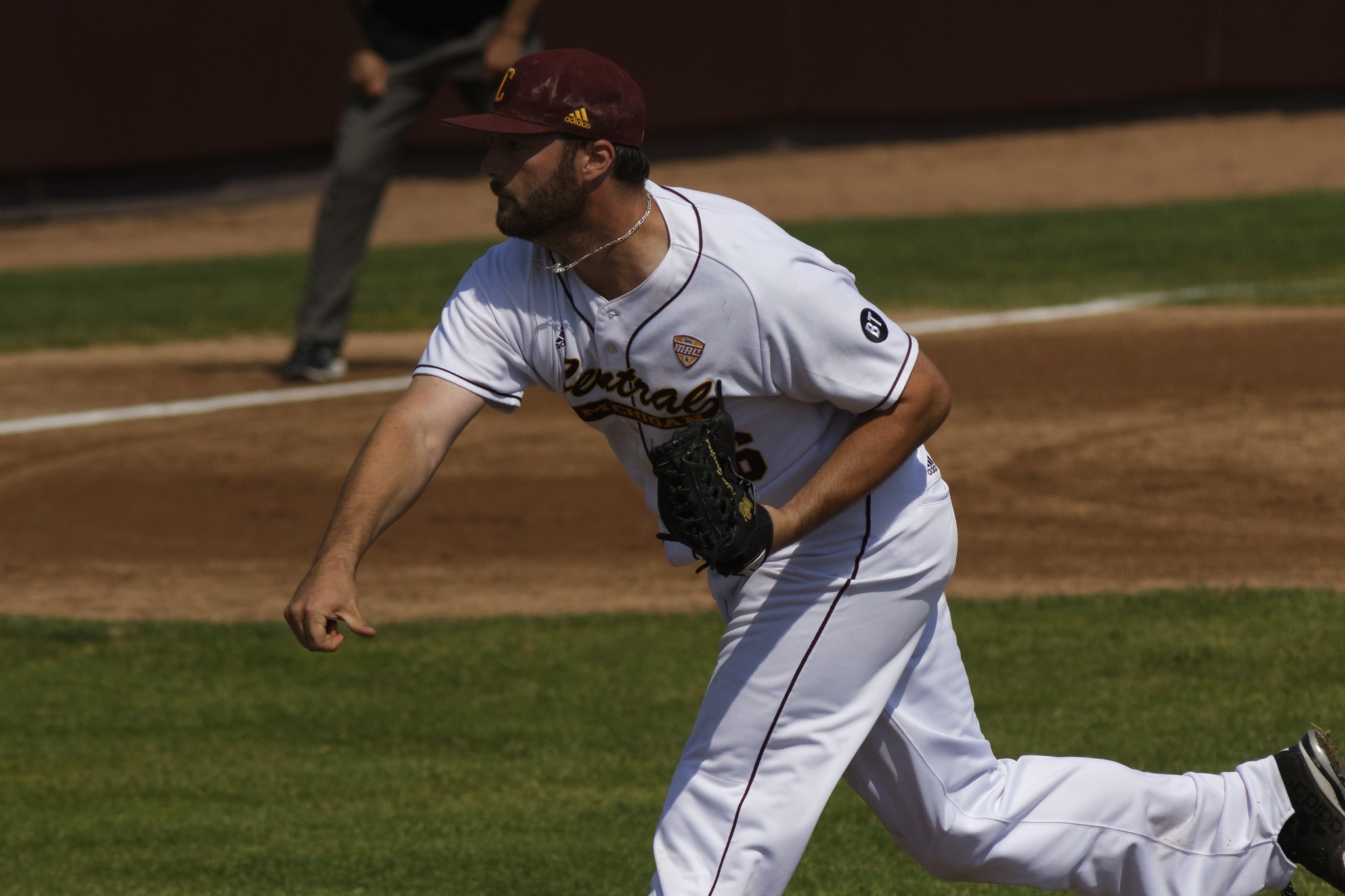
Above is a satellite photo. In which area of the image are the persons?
[268,0,554,382]
[280,48,1345,896]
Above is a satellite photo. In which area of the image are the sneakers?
[1270,729,1345,896]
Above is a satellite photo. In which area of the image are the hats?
[435,46,647,153]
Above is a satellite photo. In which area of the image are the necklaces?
[534,190,652,273]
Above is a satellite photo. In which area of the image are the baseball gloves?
[648,411,773,579]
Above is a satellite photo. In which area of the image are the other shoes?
[282,338,344,379]
